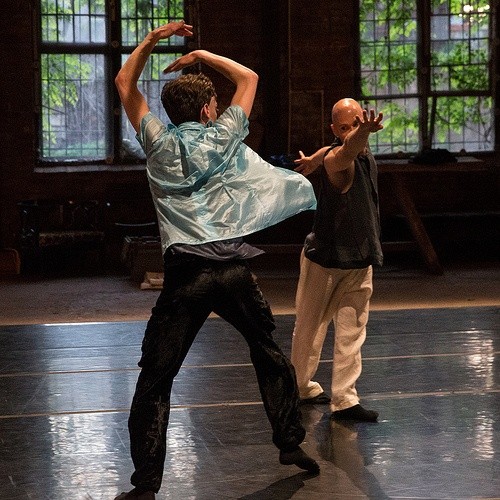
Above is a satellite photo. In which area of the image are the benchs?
[16,197,106,270]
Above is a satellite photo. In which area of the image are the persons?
[290,97,384,418]
[114,20,316,500]
